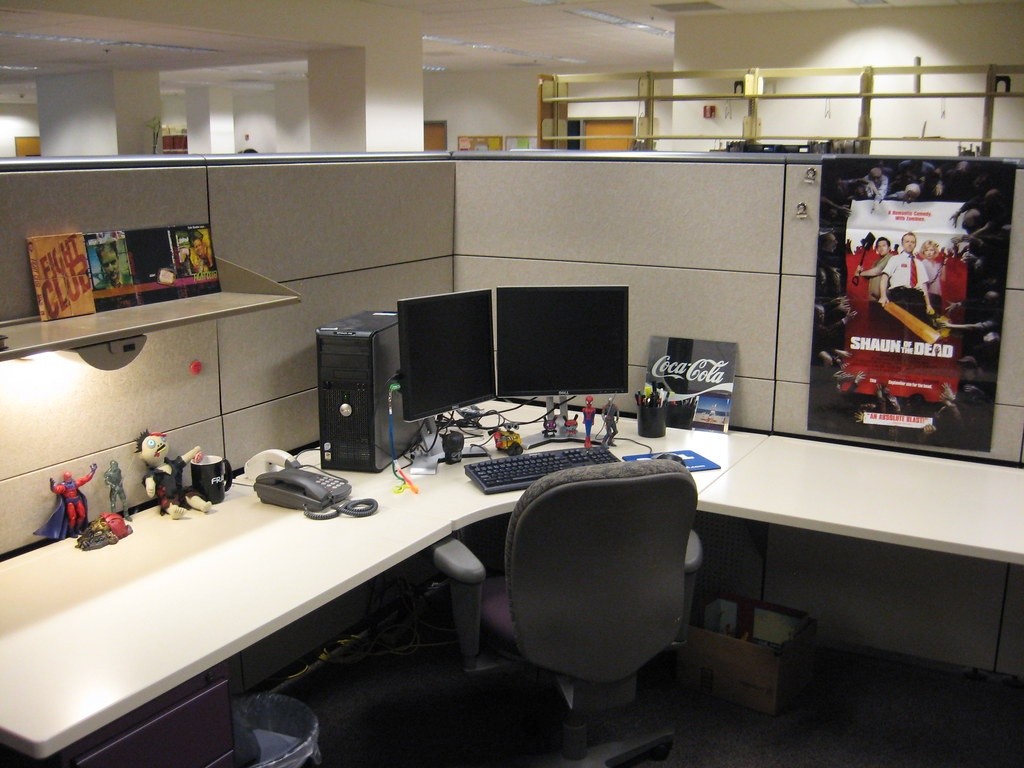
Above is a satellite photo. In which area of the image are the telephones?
[252,468,352,512]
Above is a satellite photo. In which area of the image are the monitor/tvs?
[496,286,629,449]
[396,288,496,475]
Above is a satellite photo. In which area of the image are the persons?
[93,238,131,290]
[104,459,132,522]
[134,429,212,520]
[183,239,212,277]
[811,160,1012,446]
[33,462,98,540]
[601,397,619,448]
[583,396,595,448]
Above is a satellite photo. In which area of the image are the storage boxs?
[677,593,820,717]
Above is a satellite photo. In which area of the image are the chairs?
[426,464,705,768]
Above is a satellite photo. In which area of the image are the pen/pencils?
[634,380,670,408]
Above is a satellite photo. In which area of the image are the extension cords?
[381,596,426,645]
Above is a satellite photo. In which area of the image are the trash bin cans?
[231,692,320,768]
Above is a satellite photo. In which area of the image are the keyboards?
[464,447,622,495]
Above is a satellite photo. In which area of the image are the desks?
[0,158,1024,768]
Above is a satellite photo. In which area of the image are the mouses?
[651,454,686,468]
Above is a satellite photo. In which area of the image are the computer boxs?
[316,310,429,473]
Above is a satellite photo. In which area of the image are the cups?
[191,455,232,504]
[636,404,666,437]
